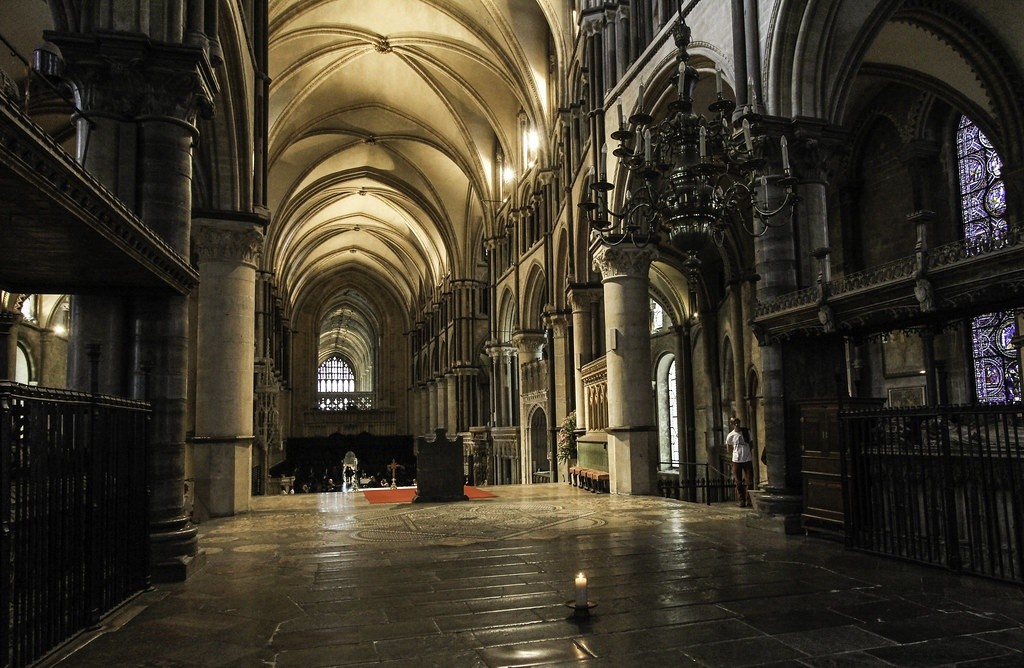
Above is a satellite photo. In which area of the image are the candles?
[565,572,597,618]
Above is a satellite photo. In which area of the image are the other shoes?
[739,499,752,507]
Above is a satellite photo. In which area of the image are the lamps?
[575,1,796,294]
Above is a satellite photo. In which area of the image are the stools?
[567,466,609,495]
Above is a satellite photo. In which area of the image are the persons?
[725,418,754,507]
[279,466,418,494]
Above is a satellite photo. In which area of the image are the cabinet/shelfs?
[792,396,889,540]
